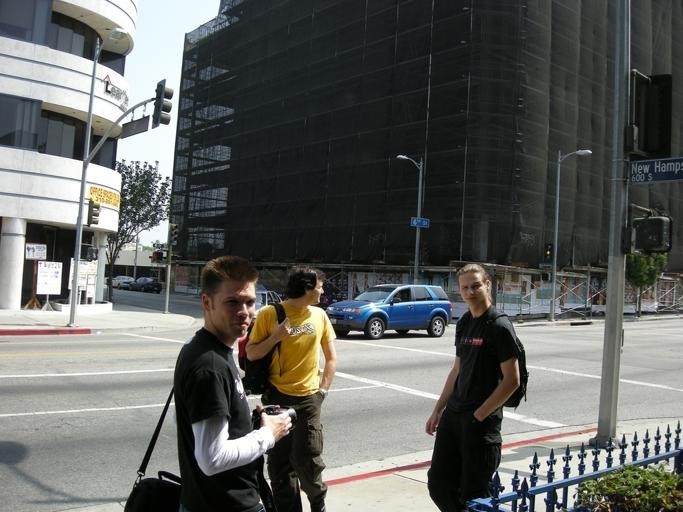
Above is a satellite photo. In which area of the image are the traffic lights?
[151,79,174,129]
[544,242,553,263]
[169,223,178,242]
[87,200,101,224]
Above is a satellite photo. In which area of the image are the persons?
[393,292,405,304]
[598,289,606,316]
[174,255,293,510]
[591,288,599,315]
[326,279,339,304]
[246,268,336,512]
[425,264,521,511]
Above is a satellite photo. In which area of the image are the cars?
[112,275,135,290]
[247,290,283,332]
[128,277,163,294]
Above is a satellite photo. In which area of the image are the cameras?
[251,406,298,432]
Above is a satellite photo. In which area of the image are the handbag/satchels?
[126,470,181,507]
[238,300,287,389]
[482,310,528,409]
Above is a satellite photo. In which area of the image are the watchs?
[320,387,328,398]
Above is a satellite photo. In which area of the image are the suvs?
[326,283,454,341]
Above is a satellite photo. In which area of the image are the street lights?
[397,154,424,284]
[548,149,593,322]
[65,25,128,328]
